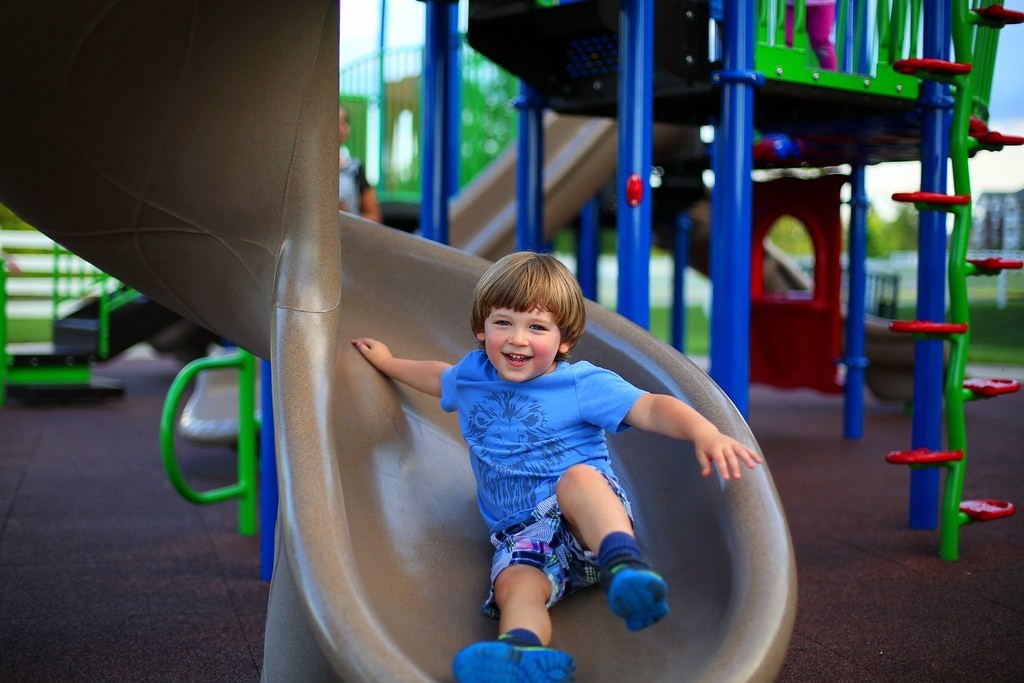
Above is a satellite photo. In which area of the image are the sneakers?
[452,634,576,683]
[602,557,670,634]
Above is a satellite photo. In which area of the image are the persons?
[339,106,382,224]
[785,0,838,72]
[349,251,764,682]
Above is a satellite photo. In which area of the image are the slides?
[1,0,802,683]
[651,189,949,403]
[176,112,689,450]
